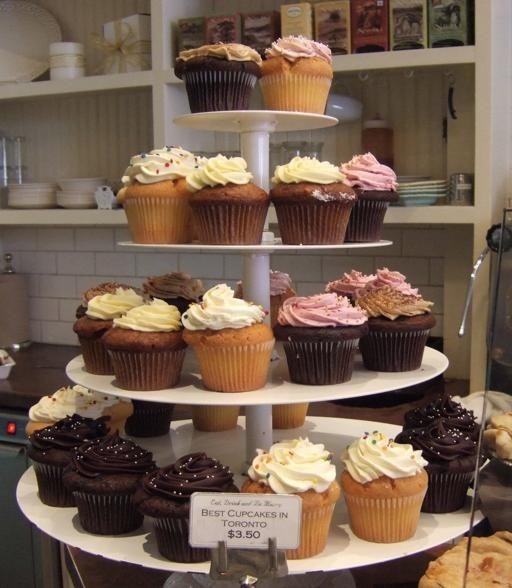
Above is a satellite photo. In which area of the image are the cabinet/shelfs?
[1,1,494,226]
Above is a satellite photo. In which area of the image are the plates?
[390,173,452,206]
[8,176,110,209]
[0,1,64,84]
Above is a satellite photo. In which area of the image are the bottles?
[361,111,394,171]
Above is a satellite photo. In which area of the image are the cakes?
[174,39,264,113]
[116,143,399,245]
[259,33,333,115]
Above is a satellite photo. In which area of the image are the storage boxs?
[97,9,150,74]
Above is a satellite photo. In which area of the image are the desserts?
[25,269,482,392]
[27,385,482,564]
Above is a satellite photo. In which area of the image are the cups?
[179,69,258,113]
[0,135,28,187]
[450,172,472,204]
[49,42,88,82]
[191,138,323,182]
[254,72,332,116]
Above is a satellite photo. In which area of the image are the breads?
[483,414,512,462]
[417,530,512,588]
